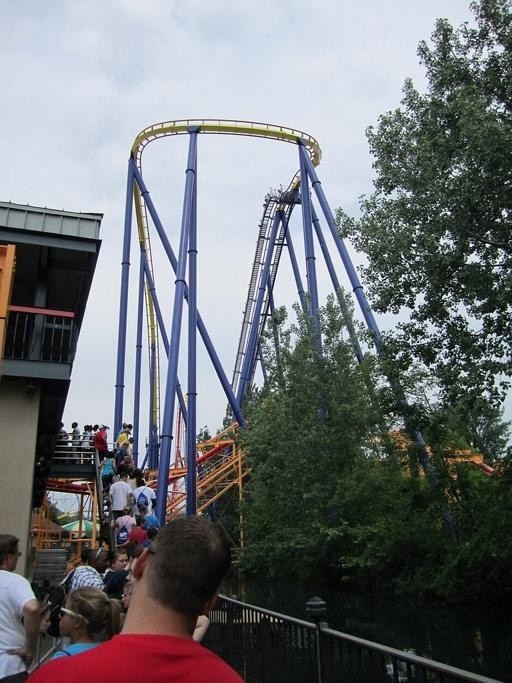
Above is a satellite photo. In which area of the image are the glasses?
[59,605,88,624]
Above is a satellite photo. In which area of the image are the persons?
[57,421,109,459]
[192,593,218,641]
[60,423,161,625]
[0,532,42,682]
[50,588,121,662]
[29,516,245,682]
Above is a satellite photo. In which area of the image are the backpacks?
[43,568,76,638]
[117,516,132,544]
[137,486,148,508]
